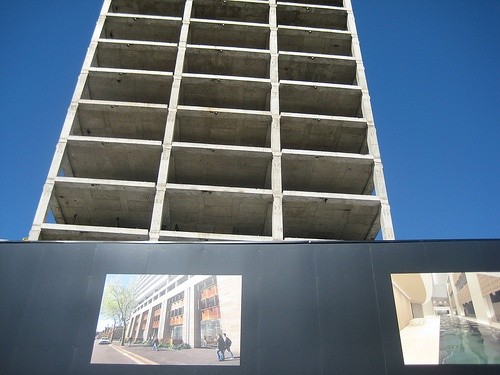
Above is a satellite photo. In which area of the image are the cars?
[99,337,109,344]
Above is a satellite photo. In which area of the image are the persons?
[127,336,208,352]
[216,334,224,361]
[223,333,234,359]
